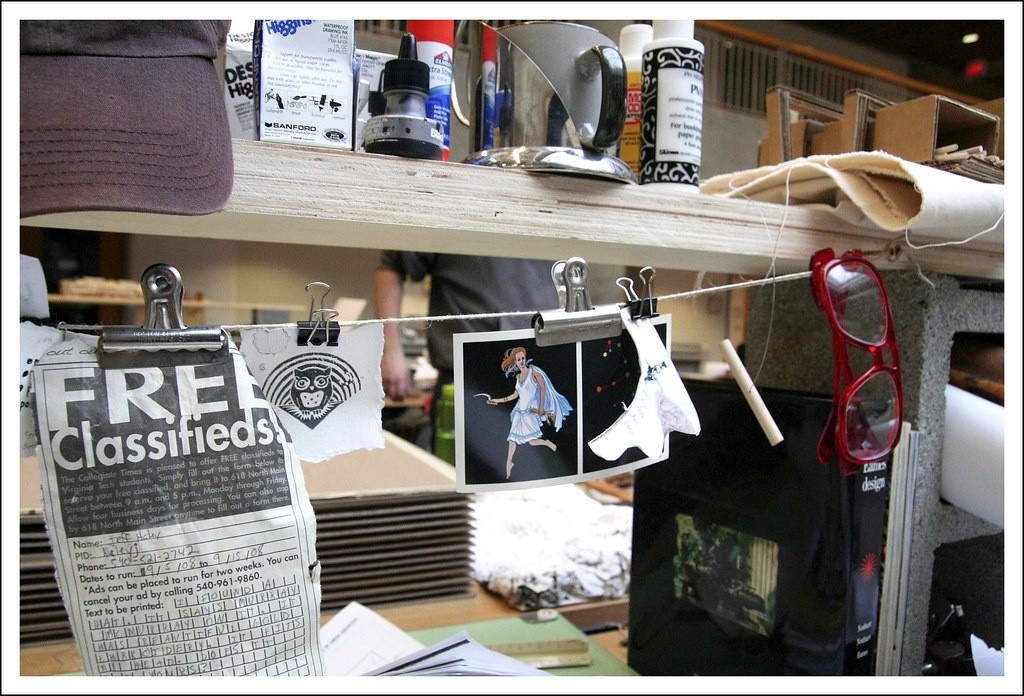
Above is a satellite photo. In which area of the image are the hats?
[19,20,236,218]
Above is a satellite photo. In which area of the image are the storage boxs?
[755,87,1003,182]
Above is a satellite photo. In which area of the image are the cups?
[464,23,639,182]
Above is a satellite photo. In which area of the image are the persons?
[372,249,561,457]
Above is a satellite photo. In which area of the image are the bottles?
[435,385,455,464]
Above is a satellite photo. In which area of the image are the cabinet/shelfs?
[19,20,1004,678]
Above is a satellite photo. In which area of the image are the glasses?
[810,246,904,477]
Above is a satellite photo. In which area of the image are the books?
[623,372,921,675]
